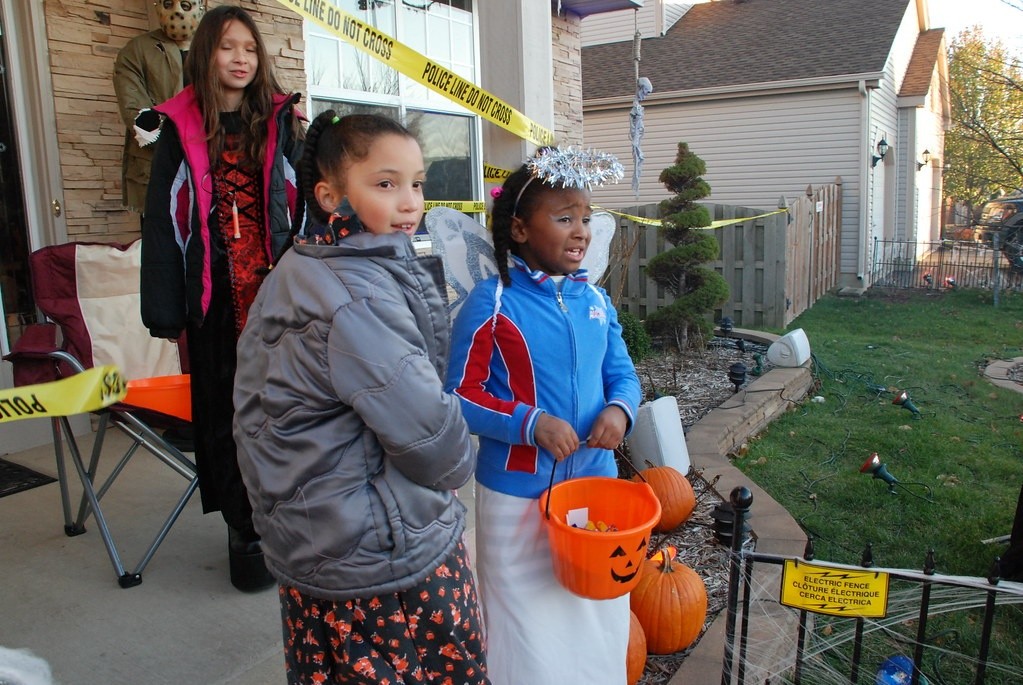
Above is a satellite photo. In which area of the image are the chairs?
[8,232,205,590]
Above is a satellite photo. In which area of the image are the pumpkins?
[630,548,707,654]
[631,459,696,533]
[626,610,646,685]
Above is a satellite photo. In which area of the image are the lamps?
[918,149,931,171]
[946,278,957,288]
[862,454,901,495]
[922,272,933,289]
[892,390,922,420]
[872,134,891,167]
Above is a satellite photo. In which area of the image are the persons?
[443,146,644,685]
[231,108,489,684]
[113,0,207,235]
[140,4,366,592]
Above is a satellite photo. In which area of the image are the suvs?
[973,194,1023,271]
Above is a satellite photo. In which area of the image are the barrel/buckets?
[538,437,662,602]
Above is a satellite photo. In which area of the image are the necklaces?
[218,137,241,238]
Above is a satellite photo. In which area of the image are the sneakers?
[223,505,261,542]
